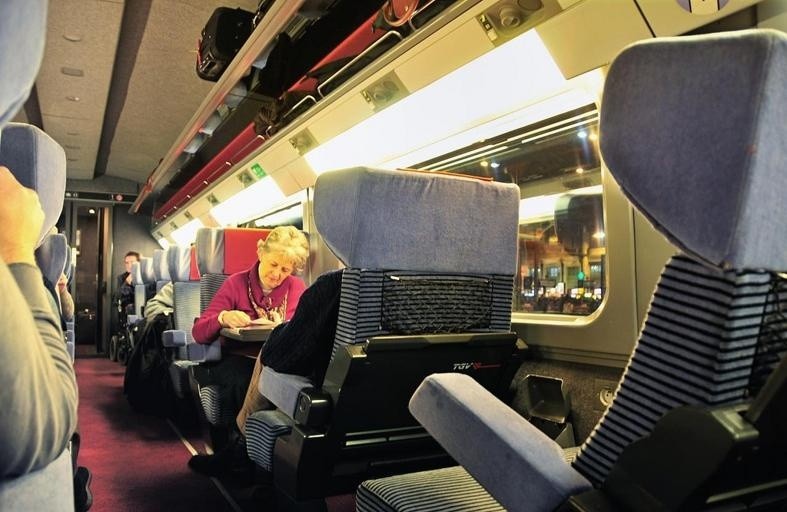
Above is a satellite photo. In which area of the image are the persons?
[188,268,347,481]
[0,166,94,512]
[192,226,312,423]
[117,250,139,306]
[143,283,173,323]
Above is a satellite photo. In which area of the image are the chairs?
[117,164,532,512]
[0,0,103,512]
[345,23,786,512]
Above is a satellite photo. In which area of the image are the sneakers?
[73,465,94,512]
[187,451,257,490]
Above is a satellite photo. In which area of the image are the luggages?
[194,6,268,81]
[247,0,323,56]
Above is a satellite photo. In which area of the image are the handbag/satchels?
[122,311,177,422]
